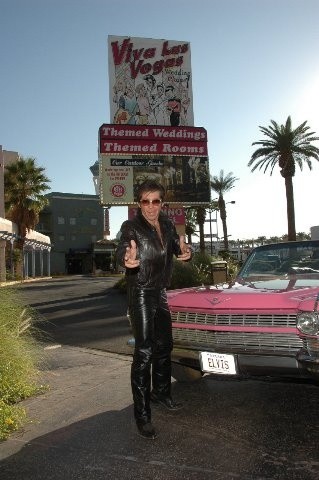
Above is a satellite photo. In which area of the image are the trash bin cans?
[211,260,227,286]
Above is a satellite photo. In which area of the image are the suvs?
[263,255,281,268]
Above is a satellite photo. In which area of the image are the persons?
[115,180,195,440]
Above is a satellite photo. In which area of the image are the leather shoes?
[151,391,183,411]
[136,420,156,438]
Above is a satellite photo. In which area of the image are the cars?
[128,240,319,377]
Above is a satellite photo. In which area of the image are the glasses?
[139,199,162,206]
